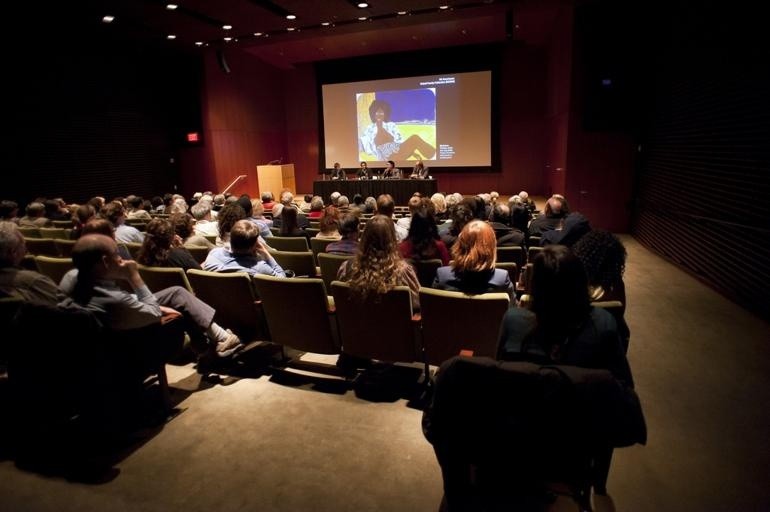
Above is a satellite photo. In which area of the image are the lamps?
[196,42,202,45]
[223,26,232,29]
[167,5,177,9]
[168,36,176,38]
[287,15,295,19]
[358,4,368,7]
[104,17,114,22]
[255,33,262,35]
[224,38,231,40]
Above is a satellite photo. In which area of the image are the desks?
[257,164,296,201]
[313,180,438,207]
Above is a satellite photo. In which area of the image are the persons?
[335,196,361,217]
[163,193,188,213]
[249,199,274,227]
[349,194,365,210]
[409,160,430,180]
[538,212,591,245]
[571,229,626,300]
[432,190,462,204]
[215,204,276,251]
[396,197,422,230]
[412,198,452,233]
[432,219,519,308]
[136,218,203,270]
[326,214,361,256]
[376,193,401,240]
[300,193,313,210]
[204,220,286,277]
[302,196,322,213]
[329,192,341,208]
[279,206,311,249]
[528,198,574,236]
[498,246,615,367]
[60,233,246,357]
[272,203,313,237]
[369,100,435,160]
[213,193,236,204]
[485,204,510,233]
[382,161,400,180]
[71,197,127,218]
[308,199,325,217]
[399,207,450,266]
[261,191,276,209]
[336,214,421,310]
[237,197,273,238]
[463,191,499,204]
[127,194,163,219]
[0,197,69,223]
[169,214,217,252]
[76,218,119,260]
[1,221,84,312]
[316,206,343,239]
[281,192,304,214]
[331,163,346,180]
[356,162,372,179]
[508,191,528,218]
[191,192,213,219]
[360,197,377,214]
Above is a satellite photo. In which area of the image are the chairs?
[264,210,272,212]
[358,223,366,230]
[267,251,318,278]
[187,270,258,369]
[405,260,442,287]
[302,209,310,213]
[36,254,77,286]
[529,236,541,247]
[51,221,72,229]
[305,228,320,237]
[393,219,398,224]
[265,216,272,220]
[417,358,607,511]
[496,247,521,272]
[22,255,37,273]
[269,228,280,235]
[495,263,518,290]
[331,281,416,389]
[303,214,310,217]
[357,230,364,239]
[528,247,542,263]
[519,295,631,345]
[306,218,320,222]
[186,246,215,265]
[263,213,273,216]
[1,297,182,460]
[65,229,79,239]
[255,274,338,381]
[263,236,308,252]
[16,227,40,238]
[309,222,320,228]
[122,242,145,262]
[418,287,515,402]
[202,236,217,246]
[40,228,64,239]
[493,228,512,239]
[356,213,376,224]
[317,253,359,285]
[54,239,77,257]
[23,238,54,254]
[130,223,147,232]
[311,238,340,259]
[133,263,194,297]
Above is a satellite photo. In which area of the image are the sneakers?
[217,362,254,384]
[216,326,254,357]
